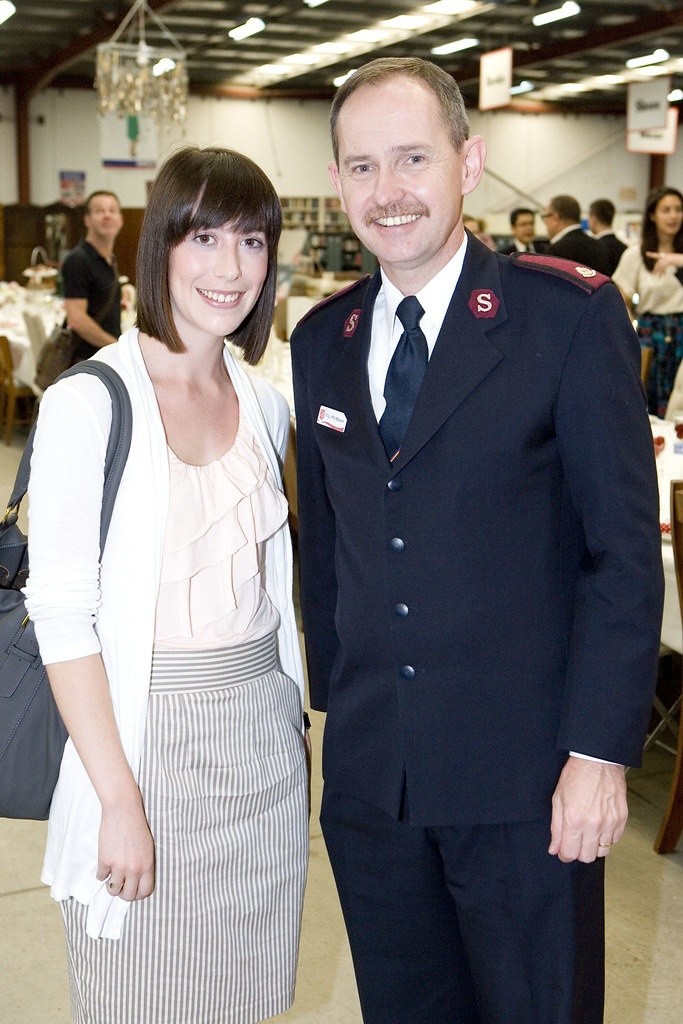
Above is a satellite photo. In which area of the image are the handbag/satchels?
[34,324,79,390]
[0,362,132,820]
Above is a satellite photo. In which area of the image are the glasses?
[541,213,553,219]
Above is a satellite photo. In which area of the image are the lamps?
[94,0,189,121]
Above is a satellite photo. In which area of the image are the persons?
[58,190,126,364]
[23,147,314,1024]
[290,57,665,1024]
[461,185,683,422]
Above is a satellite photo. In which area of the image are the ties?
[526,245,530,253]
[378,296,429,467]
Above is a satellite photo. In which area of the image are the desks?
[623,414,683,781]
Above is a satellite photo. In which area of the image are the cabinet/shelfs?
[278,199,364,338]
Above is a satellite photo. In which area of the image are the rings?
[599,843,613,848]
[110,882,123,890]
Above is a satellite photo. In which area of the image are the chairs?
[654,480,683,855]
[0,337,37,446]
